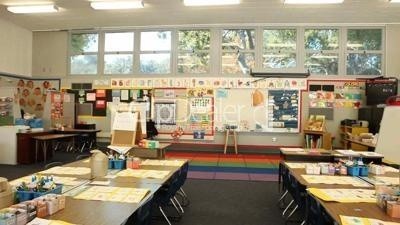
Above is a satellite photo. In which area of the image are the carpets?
[161,151,289,182]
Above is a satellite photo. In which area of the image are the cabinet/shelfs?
[303,130,331,150]
[338,125,369,151]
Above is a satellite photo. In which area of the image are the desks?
[0,122,193,225]
[277,146,400,225]
